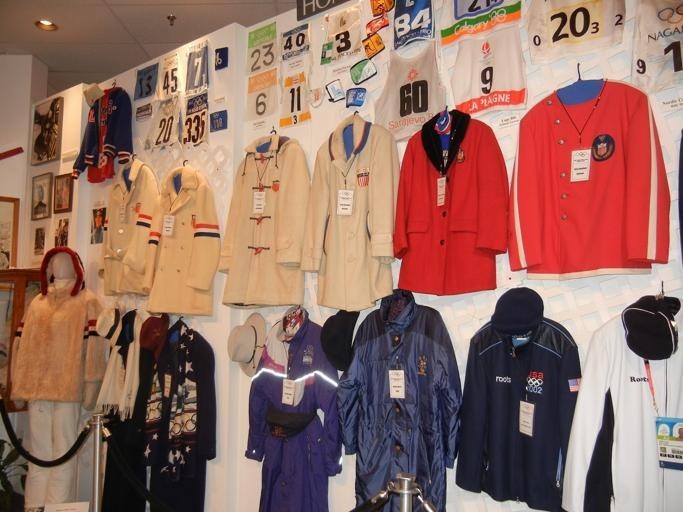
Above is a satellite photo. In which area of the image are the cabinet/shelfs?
[0,269,41,397]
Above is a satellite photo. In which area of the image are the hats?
[94,304,123,346]
[227,312,267,376]
[140,314,170,361]
[491,287,544,340]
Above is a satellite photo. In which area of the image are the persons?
[94,211,103,243]
[33,185,46,215]
[9,247,106,512]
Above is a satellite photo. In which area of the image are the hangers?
[110,82,118,88]
[131,154,137,160]
[660,280,665,295]
[257,129,276,148]
[444,104,448,114]
[182,159,188,166]
[576,62,582,80]
[178,316,183,322]
[353,109,359,117]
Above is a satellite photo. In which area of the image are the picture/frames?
[54,173,72,213]
[30,173,52,221]
[31,96,64,164]
[1,195,20,269]
